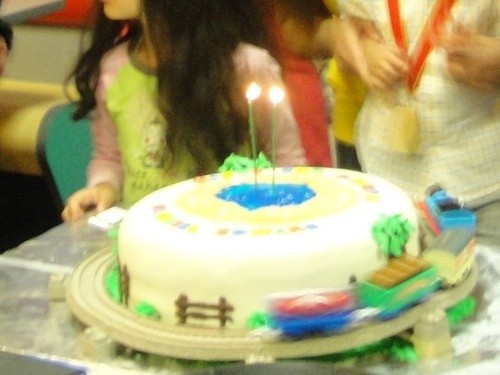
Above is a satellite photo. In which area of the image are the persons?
[247,2,381,167]
[328,49,372,175]
[334,0,499,250]
[61,1,308,222]
[0,18,13,77]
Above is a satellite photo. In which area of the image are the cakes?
[106,152,421,334]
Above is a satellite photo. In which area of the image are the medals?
[387,1,453,159]
